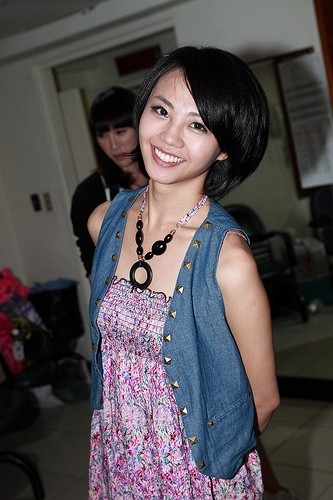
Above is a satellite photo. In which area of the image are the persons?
[70,86,148,283]
[86,46,281,500]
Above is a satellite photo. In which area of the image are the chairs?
[224,203,309,325]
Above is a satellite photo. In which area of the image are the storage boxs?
[293,237,328,283]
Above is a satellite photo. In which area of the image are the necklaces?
[129,186,208,289]
